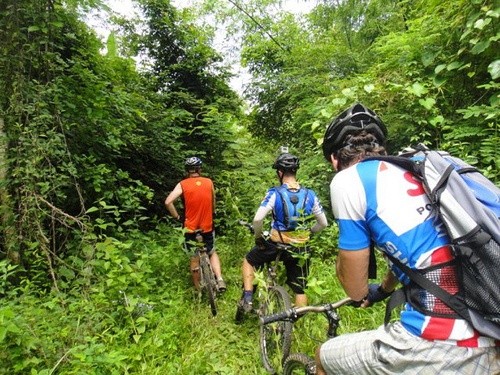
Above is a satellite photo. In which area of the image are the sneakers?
[216,281,225,297]
[235,298,253,322]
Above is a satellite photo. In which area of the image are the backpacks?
[361,149,500,339]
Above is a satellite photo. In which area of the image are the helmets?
[323,103,387,162]
[185,157,202,166]
[272,153,300,168]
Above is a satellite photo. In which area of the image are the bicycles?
[259,294,356,375]
[237,220,293,374]
[179,217,226,317]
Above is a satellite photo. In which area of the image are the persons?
[164,157,228,302]
[241,152,327,312]
[314,100,500,375]
